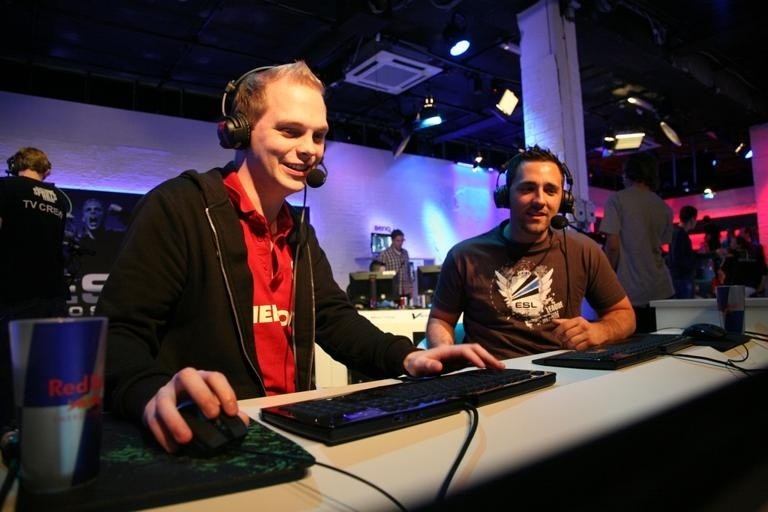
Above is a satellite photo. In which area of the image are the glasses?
[395,238,406,243]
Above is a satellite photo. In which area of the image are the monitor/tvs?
[349,271,401,305]
[417,265,440,293]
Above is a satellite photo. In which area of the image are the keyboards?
[260,368,554,446]
[533,334,694,370]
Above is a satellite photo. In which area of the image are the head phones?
[493,150,574,214]
[217,66,331,150]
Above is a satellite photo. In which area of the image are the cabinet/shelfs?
[649,297,767,335]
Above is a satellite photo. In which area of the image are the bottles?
[369,275,377,309]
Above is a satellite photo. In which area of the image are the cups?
[714,286,747,333]
[9,318,108,481]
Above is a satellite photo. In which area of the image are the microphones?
[305,158,332,188]
[551,216,574,230]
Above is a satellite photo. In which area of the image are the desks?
[9,324,766,512]
[314,308,432,388]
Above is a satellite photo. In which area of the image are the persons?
[369,229,413,308]
[0,148,71,426]
[598,154,768,332]
[82,197,132,273]
[95,61,505,454]
[426,146,636,351]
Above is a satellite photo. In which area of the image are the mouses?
[684,322,730,345]
[175,401,249,458]
